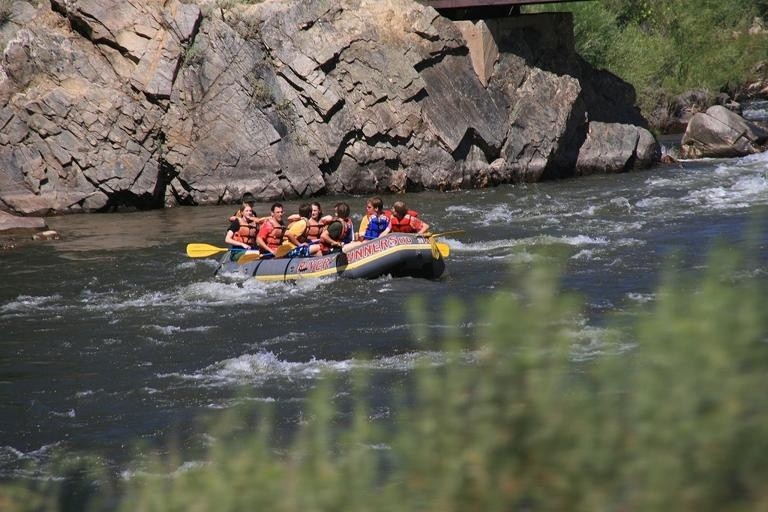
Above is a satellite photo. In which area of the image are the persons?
[225,202,260,250]
[228,197,257,223]
[318,202,354,256]
[288,201,333,252]
[283,204,320,256]
[355,196,391,242]
[254,203,288,258]
[378,200,430,238]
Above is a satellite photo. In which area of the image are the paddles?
[187,243,248,258]
[437,244,449,257]
[237,254,274,265]
[276,241,320,258]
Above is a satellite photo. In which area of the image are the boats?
[210,229,447,288]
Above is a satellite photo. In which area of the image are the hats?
[243,196,257,203]
